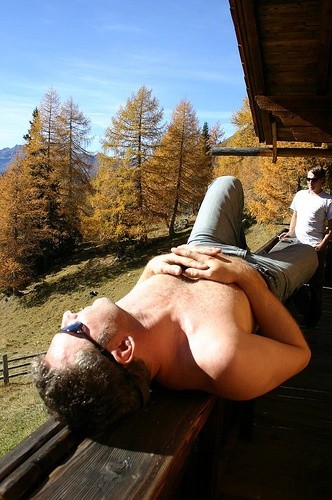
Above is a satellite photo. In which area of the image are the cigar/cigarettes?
[277,235,282,238]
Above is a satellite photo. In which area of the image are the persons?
[278,166,332,328]
[32,176,319,437]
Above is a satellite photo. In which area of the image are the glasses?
[56,321,122,367]
[306,178,319,182]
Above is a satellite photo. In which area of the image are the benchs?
[0,228,311,500]
